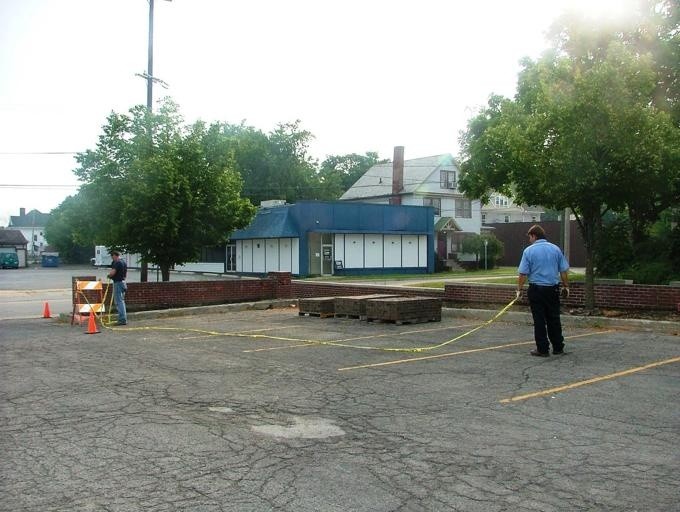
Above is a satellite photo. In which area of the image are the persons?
[109,251,128,326]
[516,224,570,357]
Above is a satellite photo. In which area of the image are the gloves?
[517,290,524,302]
[560,287,570,299]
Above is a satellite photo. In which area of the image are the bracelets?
[518,288,524,292]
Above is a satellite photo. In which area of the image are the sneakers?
[531,350,549,357]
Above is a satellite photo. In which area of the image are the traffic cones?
[84,309,101,334]
[41,302,52,318]
[71,313,87,326]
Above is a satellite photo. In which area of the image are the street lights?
[484,239,489,270]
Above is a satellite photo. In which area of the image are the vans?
[0,252,19,269]
[41,256,59,267]
